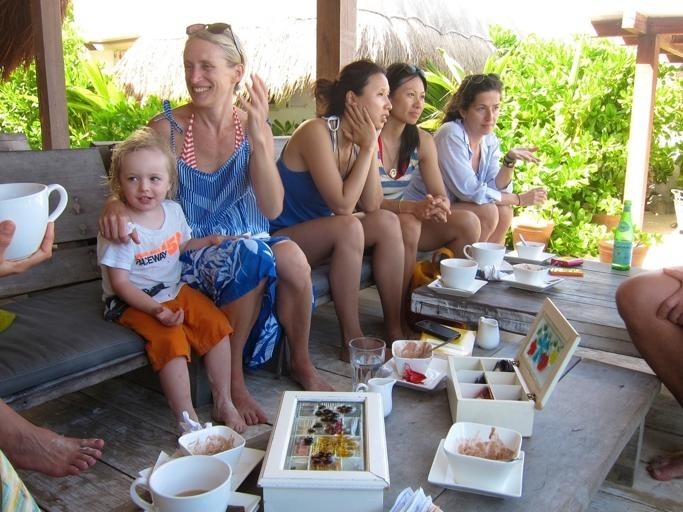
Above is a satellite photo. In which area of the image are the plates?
[146,491,262,512]
[427,437,526,501]
[168,448,267,490]
[504,249,556,264]
[426,278,488,298]
[382,357,449,392]
[500,273,563,293]
[472,260,513,270]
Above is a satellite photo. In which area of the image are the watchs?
[500,156,514,168]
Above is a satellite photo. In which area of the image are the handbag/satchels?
[408,247,471,332]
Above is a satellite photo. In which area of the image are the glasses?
[185,21,244,63]
[391,66,430,80]
[465,72,501,86]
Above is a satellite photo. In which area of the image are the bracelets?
[398,198,404,215]
[518,193,521,208]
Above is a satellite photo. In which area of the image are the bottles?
[612,200,636,273]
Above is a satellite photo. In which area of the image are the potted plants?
[593,190,624,227]
[509,151,561,250]
[594,217,662,270]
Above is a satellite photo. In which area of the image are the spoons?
[516,233,529,248]
[427,334,459,353]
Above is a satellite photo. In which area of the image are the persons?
[96,28,336,426]
[97,126,247,437]
[406,74,548,260]
[0,220,104,478]
[377,63,481,342]
[269,59,414,363]
[614,266,683,480]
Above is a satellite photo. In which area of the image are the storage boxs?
[257,391,390,512]
[447,297,581,438]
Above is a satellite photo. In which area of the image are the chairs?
[0,134,446,413]
[670,188,683,236]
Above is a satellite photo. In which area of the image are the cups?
[131,454,231,512]
[515,240,545,258]
[356,375,397,417]
[440,257,478,289]
[476,317,501,350]
[348,338,386,395]
[464,241,506,270]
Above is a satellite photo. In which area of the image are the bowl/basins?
[514,264,549,285]
[178,424,246,471]
[443,421,521,489]
[391,340,433,377]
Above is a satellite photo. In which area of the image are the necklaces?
[381,134,401,179]
[335,120,353,180]
